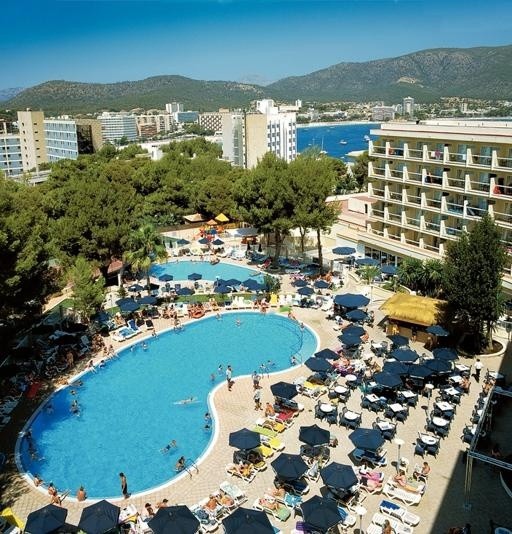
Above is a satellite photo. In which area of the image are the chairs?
[305,292,470,534]
[222,381,304,534]
[117,483,221,534]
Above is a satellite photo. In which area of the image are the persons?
[459,358,503,480]
[0,384,233,528]
[258,491,508,534]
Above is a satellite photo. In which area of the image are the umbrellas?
[0,213,231,383]
[145,504,199,534]
[77,499,121,533]
[221,507,277,533]
[24,504,69,533]
